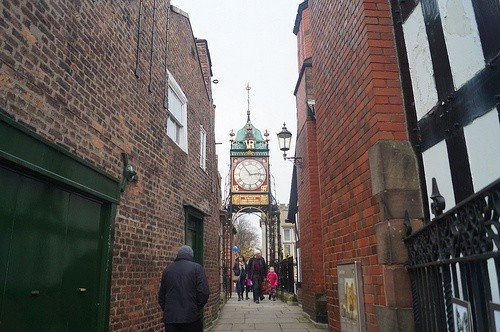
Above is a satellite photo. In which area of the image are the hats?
[254,250,260,256]
[178,246,194,257]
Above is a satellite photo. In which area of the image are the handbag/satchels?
[233,275,241,282]
[246,278,253,286]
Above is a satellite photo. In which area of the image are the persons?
[266,267,278,301]
[232,255,246,300]
[248,249,266,304]
[158,245,209,332]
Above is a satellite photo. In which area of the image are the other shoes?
[269,294,271,300]
[260,296,264,300]
[273,296,277,301]
[238,294,244,301]
[255,300,260,304]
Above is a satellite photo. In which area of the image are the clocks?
[234,159,267,191]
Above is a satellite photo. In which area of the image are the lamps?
[275,121,304,172]
